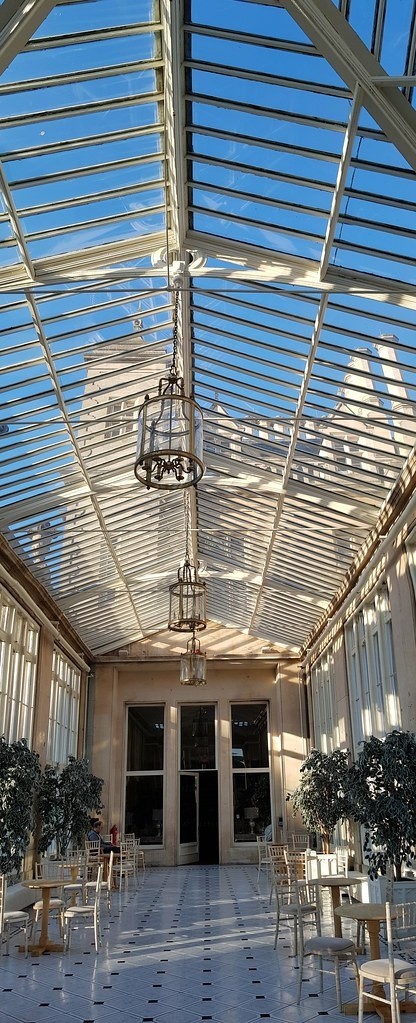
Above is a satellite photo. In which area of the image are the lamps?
[167,508,207,632]
[134,283,206,493]
[191,706,209,748]
[178,634,206,685]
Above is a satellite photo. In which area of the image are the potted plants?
[344,730,416,937]
[292,751,351,879]
[33,756,105,888]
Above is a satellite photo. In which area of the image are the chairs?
[256,831,416,1023]
[0,831,147,958]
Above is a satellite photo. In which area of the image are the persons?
[89,821,116,868]
[265,815,272,842]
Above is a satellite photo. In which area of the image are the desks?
[56,860,102,907]
[308,877,366,956]
[335,903,415,1022]
[95,853,125,889]
[278,852,316,904]
[19,879,72,952]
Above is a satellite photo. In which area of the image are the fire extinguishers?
[110,824,118,845]
